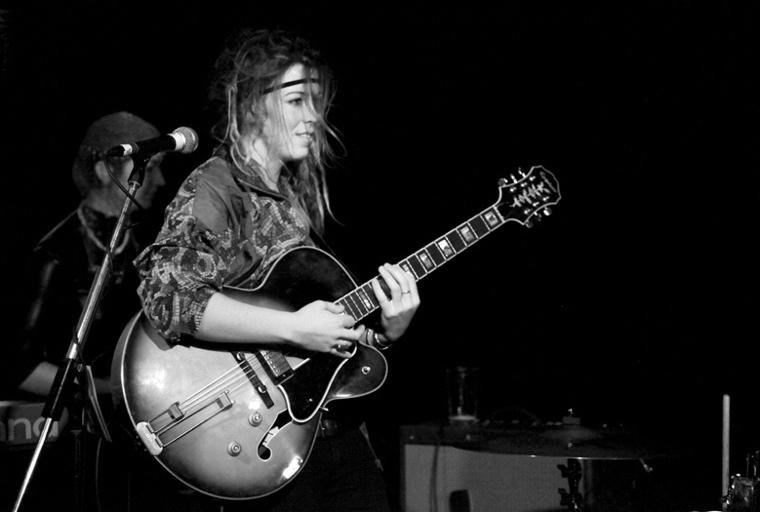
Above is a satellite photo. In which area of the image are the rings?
[337,344,343,352]
[401,291,410,294]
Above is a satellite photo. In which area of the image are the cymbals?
[396,421,534,445]
[461,423,656,466]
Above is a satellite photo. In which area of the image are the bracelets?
[366,328,372,344]
[374,332,392,347]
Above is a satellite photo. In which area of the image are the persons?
[1,110,167,510]
[133,27,421,512]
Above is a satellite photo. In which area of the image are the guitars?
[110,163,562,500]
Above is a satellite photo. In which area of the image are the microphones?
[108,127,199,157]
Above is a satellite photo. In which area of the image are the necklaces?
[74,203,135,254]
